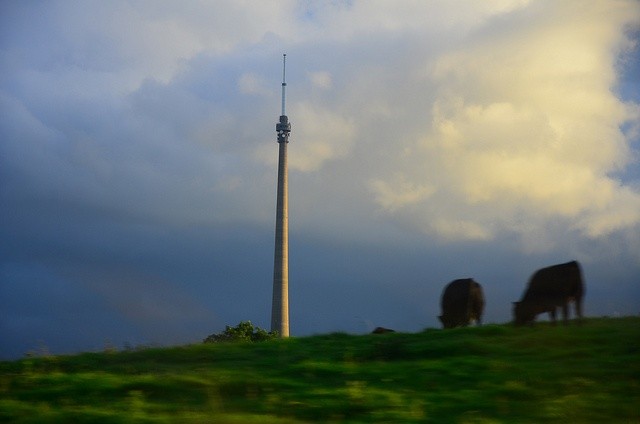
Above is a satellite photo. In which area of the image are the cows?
[437,278,486,328]
[511,260,583,326]
